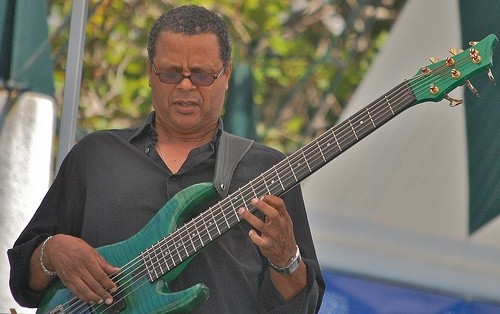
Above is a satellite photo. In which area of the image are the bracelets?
[40,236,57,276]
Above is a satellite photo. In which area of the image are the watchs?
[268,244,302,276]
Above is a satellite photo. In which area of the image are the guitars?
[36,34,500,314]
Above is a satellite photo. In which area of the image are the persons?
[7,4,327,314]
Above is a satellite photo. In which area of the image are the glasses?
[149,56,226,87]
